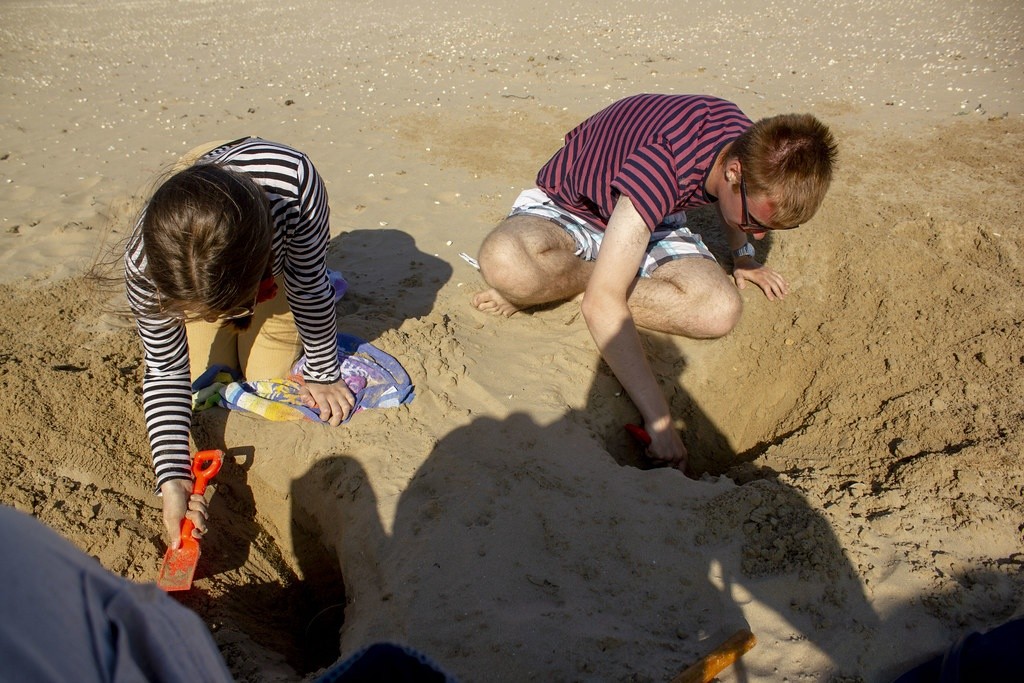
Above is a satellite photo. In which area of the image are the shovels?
[155,449,224,592]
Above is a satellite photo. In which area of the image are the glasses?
[157,250,269,319]
[738,175,765,234]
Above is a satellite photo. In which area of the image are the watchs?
[731,241,755,258]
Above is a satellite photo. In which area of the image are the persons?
[0,502,236,683]
[473,93,839,471]
[122,135,356,551]
